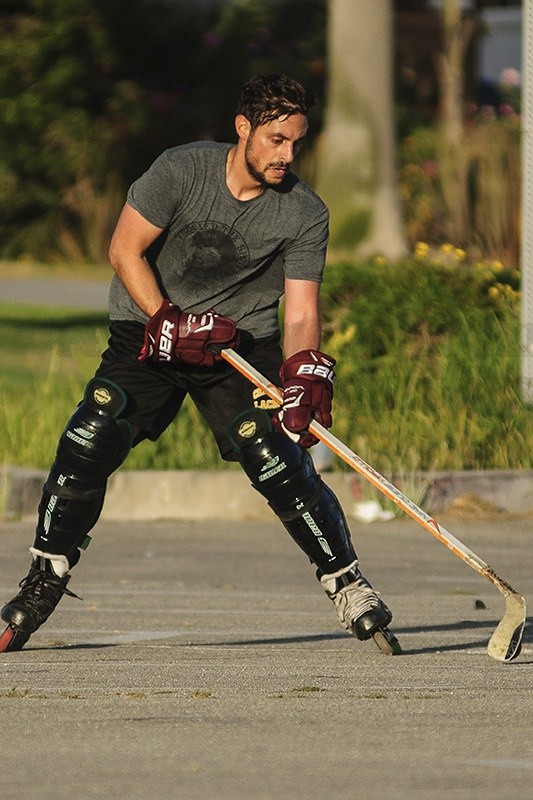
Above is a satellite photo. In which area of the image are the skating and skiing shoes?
[0,546,81,653]
[315,558,403,654]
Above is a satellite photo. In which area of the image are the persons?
[0,72,392,643]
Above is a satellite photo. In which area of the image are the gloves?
[271,350,337,448]
[136,298,241,367]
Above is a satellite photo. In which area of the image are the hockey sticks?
[217,346,527,661]
